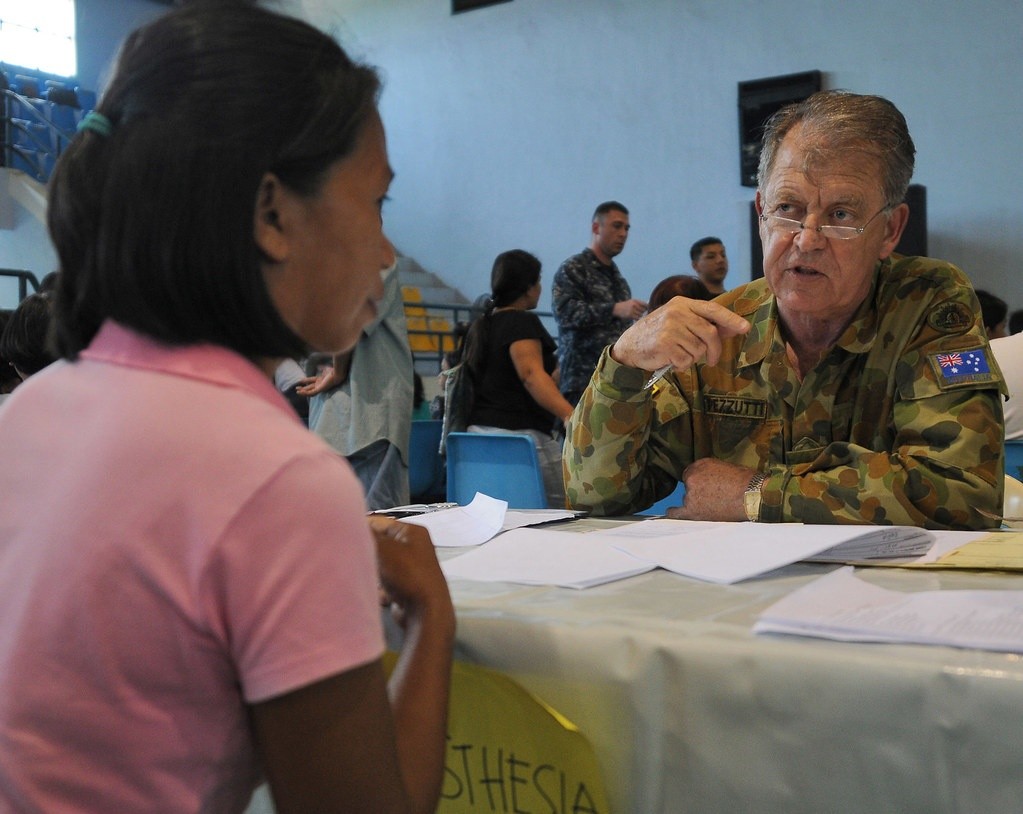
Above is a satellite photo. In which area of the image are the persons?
[0,0,1023,814]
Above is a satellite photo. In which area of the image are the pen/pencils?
[643,364,674,391]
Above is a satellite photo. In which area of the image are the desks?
[252,504,1023,814]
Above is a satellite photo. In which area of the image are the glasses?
[758,204,900,240]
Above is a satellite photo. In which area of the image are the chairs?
[401,286,428,318]
[428,318,454,352]
[445,433,546,509]
[0,66,96,183]
[409,419,443,504]
[406,317,435,351]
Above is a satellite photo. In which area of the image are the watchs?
[743,472,766,522]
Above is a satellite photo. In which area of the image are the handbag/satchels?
[438,363,461,453]
